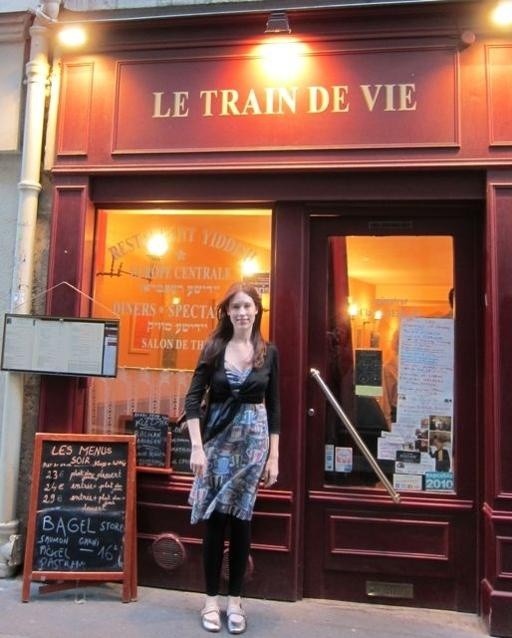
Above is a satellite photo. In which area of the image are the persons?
[441,287,453,319]
[381,327,400,423]
[414,415,451,473]
[182,281,281,637]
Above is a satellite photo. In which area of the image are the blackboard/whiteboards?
[133,411,169,467]
[24,432,136,580]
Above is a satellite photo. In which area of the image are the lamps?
[263,13,291,35]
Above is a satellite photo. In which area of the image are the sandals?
[226,602,246,634]
[200,603,222,632]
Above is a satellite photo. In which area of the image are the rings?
[273,479,277,482]
